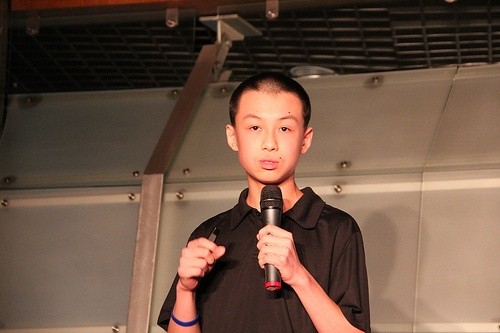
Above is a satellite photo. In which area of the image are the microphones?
[259,185,284,291]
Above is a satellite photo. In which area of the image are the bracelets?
[171,310,198,328]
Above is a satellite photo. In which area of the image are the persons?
[157,71,371,333]
